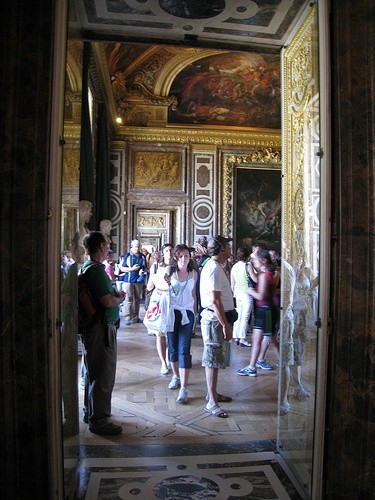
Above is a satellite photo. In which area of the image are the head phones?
[212,237,225,251]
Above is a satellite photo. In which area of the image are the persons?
[80,236,281,418]
[81,232,126,434]
[59,233,78,437]
[280,240,318,416]
